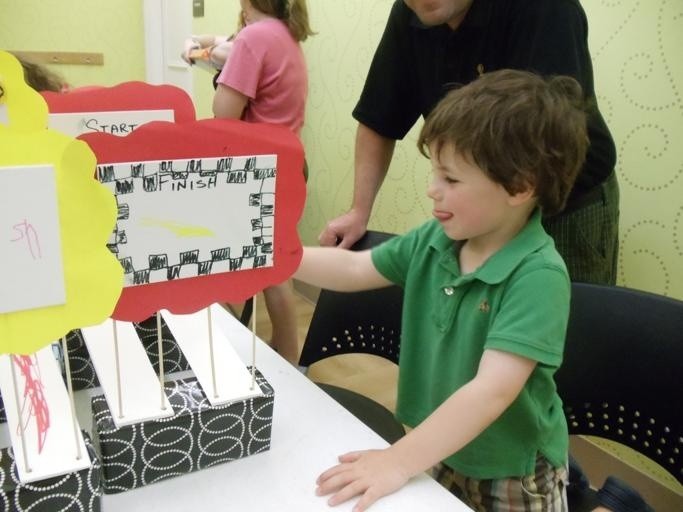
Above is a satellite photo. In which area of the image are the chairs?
[296,231,461,496]
[515,273,680,507]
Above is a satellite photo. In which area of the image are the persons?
[319,0,620,286]
[182,8,253,90]
[212,0,316,363]
[290,68,655,512]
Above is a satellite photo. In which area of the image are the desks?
[0,303,474,511]
